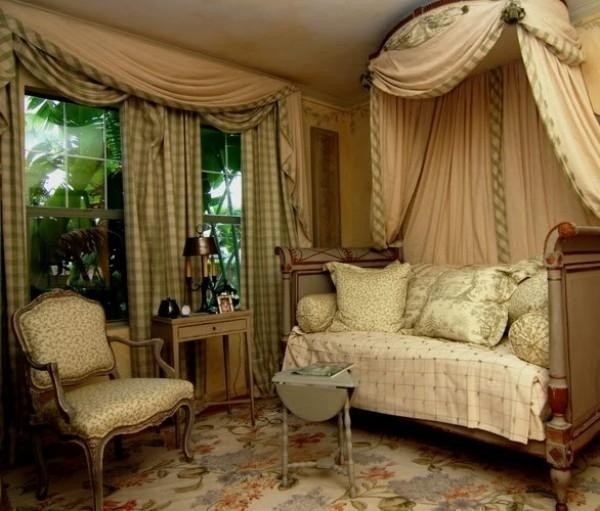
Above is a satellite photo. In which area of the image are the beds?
[275,223,600,511]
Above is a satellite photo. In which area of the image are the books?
[291,361,355,378]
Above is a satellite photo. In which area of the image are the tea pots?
[159,296,180,318]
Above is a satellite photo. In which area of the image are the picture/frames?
[216,295,233,314]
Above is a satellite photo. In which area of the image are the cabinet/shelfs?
[154,308,256,449]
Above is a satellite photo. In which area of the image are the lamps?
[182,237,219,313]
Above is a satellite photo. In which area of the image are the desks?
[272,368,359,501]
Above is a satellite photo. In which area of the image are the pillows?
[297,260,553,371]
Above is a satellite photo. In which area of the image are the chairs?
[11,288,198,510]
